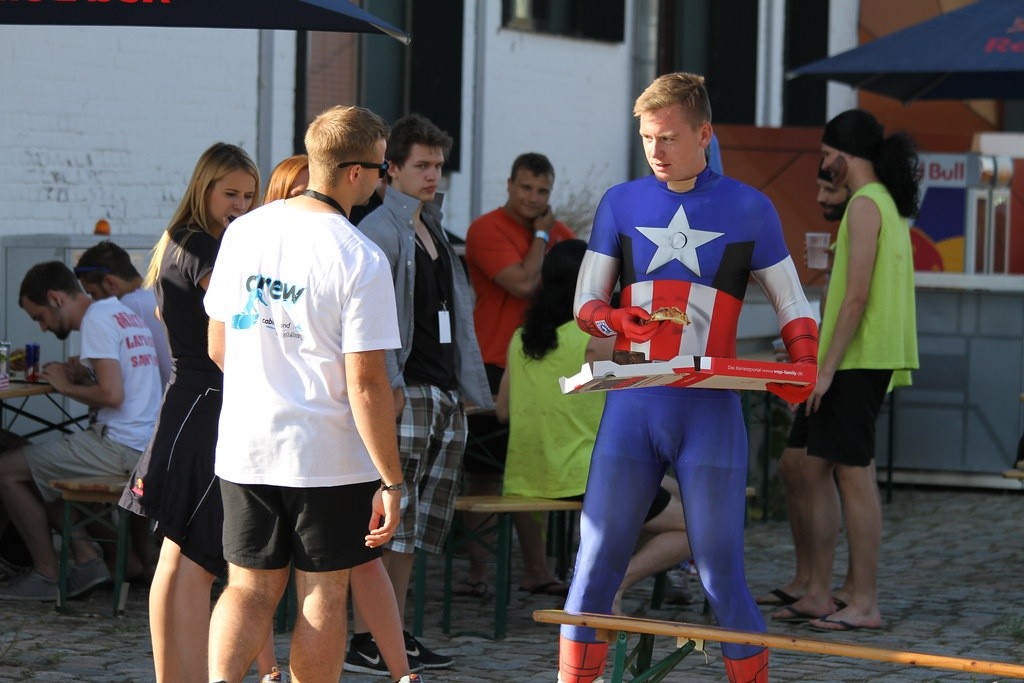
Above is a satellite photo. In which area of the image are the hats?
[817,160,833,182]
[822,109,884,159]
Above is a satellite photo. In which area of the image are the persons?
[555,69,821,683]
[756,109,920,632]
[465,153,694,619]
[118,104,496,683]
[0,242,172,603]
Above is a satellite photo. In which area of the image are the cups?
[805,232,831,270]
[24,342,40,383]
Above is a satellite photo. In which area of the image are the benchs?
[443,485,759,642]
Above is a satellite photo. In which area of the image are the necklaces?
[302,189,350,223]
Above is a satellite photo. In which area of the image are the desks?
[0,372,90,450]
[457,393,502,468]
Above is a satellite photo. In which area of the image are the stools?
[51,475,151,619]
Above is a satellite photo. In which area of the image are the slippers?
[518,580,571,594]
[754,586,803,605]
[831,596,850,612]
[451,578,486,597]
[771,606,827,621]
[806,615,882,631]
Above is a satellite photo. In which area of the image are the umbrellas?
[789,0,1024,102]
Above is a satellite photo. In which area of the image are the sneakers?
[400,631,456,668]
[344,637,425,675]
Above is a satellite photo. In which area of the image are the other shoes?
[65,557,111,597]
[664,570,692,601]
[1,566,88,602]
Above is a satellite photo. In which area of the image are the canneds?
[25,342,41,381]
[0,341,11,374]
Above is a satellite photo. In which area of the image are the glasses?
[338,158,390,179]
[74,266,111,278]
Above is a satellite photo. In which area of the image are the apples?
[94,220,109,234]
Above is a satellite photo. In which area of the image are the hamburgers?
[10,350,26,372]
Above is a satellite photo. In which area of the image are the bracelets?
[381,481,405,491]
[534,230,550,243]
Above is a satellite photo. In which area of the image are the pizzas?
[641,306,691,325]
